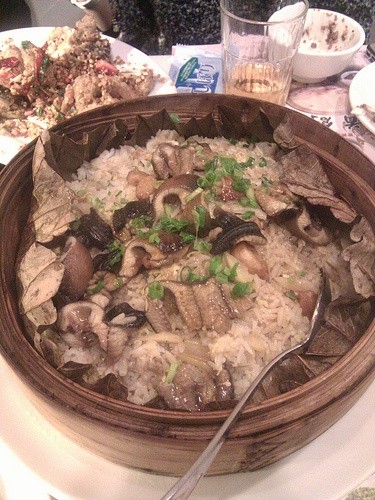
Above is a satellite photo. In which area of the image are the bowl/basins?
[267,7,365,84]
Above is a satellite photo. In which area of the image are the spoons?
[160,265,332,500]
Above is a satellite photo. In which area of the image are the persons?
[71,0,375,62]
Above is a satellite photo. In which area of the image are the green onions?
[196,156,267,220]
[148,253,250,297]
[107,205,211,271]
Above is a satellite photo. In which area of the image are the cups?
[220,0,309,107]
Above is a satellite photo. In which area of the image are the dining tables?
[0,54,375,500]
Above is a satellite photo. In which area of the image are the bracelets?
[71,0,102,10]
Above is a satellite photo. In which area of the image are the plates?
[349,62,375,135]
[0,26,178,166]
